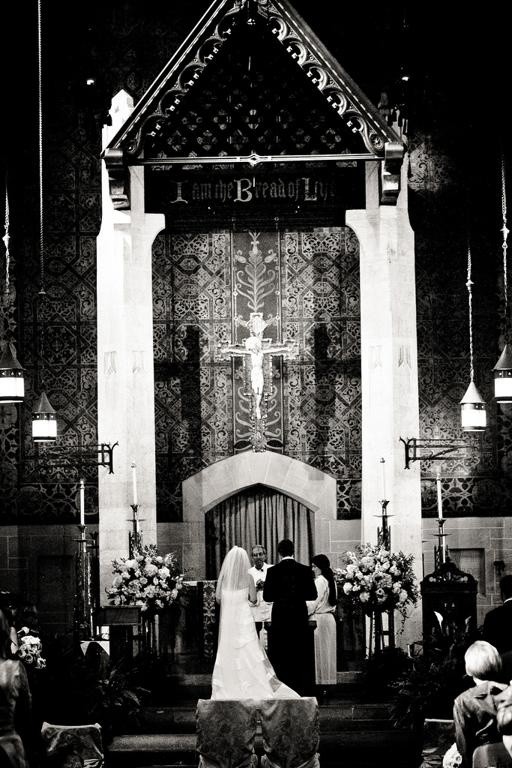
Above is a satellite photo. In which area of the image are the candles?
[436,480,443,520]
[133,465,137,505]
[79,480,88,524]
[380,457,387,500]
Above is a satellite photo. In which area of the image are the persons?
[209,545,258,709]
[248,543,276,622]
[304,553,339,704]
[262,539,317,702]
[437,571,512,767]
[0,601,164,767]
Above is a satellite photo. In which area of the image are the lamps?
[459,152,512,432]
[0,3,58,444]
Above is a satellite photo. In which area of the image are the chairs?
[40,722,104,768]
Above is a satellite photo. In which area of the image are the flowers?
[333,543,421,618]
[104,542,185,616]
[19,634,47,669]
